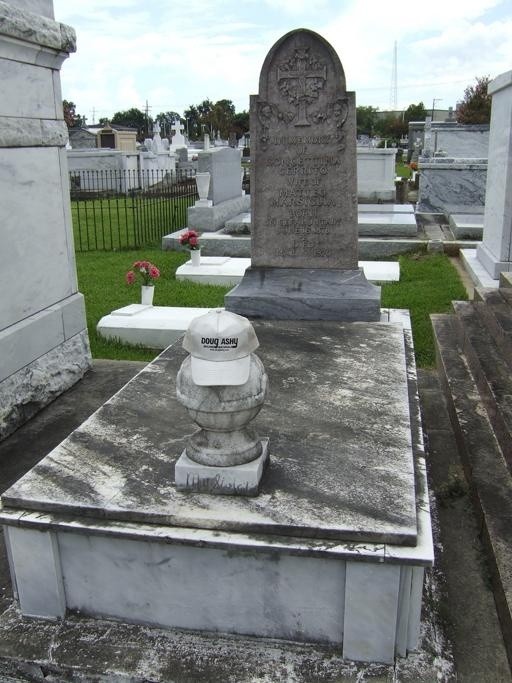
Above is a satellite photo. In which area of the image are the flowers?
[179,229,202,249]
[126,261,161,286]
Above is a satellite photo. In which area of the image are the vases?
[191,250,200,267]
[141,286,155,305]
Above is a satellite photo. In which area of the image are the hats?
[182,308,260,386]
